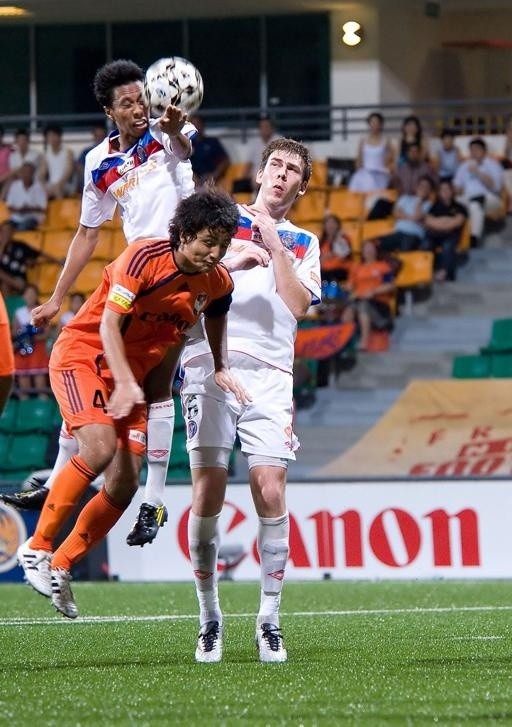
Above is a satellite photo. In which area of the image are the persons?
[1,301,17,423]
[174,137,322,662]
[2,58,196,547]
[18,188,254,619]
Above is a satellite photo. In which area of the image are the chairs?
[0,134,512,484]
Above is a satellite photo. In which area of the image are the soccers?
[139,53,206,118]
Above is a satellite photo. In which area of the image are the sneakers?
[48,568,78,619]
[17,534,51,596]
[253,613,287,661]
[124,500,168,545]
[194,619,224,663]
[0,485,50,512]
